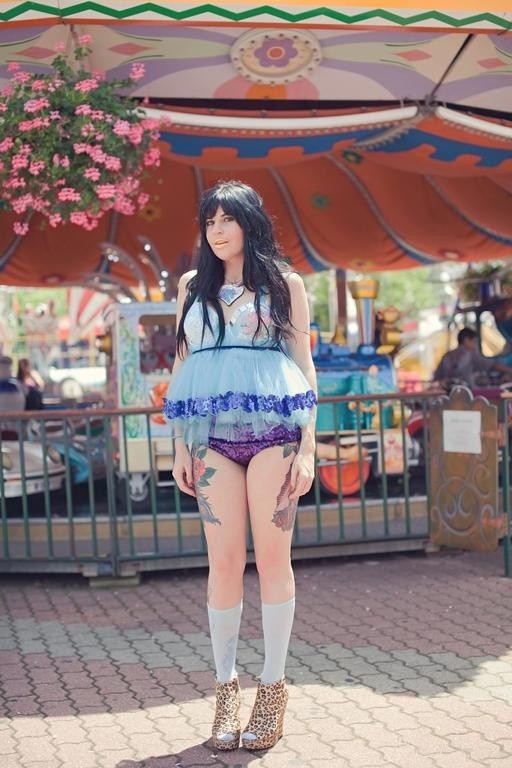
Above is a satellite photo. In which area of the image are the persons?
[165,181,317,758]
[316,436,368,466]
[431,326,512,382]
[0,356,40,393]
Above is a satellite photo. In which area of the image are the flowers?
[2,34,170,238]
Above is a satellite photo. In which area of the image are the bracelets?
[169,434,185,439]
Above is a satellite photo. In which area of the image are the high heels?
[211,675,289,751]
